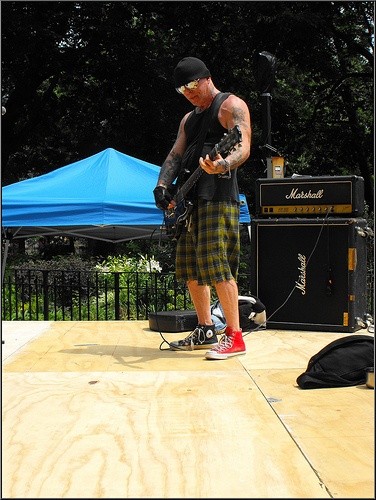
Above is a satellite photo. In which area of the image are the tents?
[0,148,253,282]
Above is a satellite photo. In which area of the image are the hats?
[172,57,211,88]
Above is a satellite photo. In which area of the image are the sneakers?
[205,327,246,359]
[169,324,218,350]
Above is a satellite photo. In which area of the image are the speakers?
[254,216,366,333]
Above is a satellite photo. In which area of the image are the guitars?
[161,124,244,243]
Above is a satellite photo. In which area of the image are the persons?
[153,56,252,361]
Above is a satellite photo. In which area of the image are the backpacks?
[296,335,375,388]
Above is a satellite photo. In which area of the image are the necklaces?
[198,91,221,112]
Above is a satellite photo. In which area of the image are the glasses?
[175,78,201,94]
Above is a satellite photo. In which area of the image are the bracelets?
[216,160,232,179]
